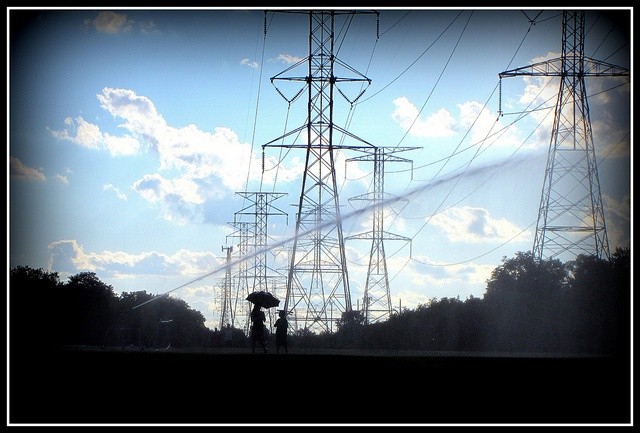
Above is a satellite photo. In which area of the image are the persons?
[251,303,270,352]
[274,310,289,354]
[214,324,234,347]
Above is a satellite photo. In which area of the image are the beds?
[245,290,281,310]
